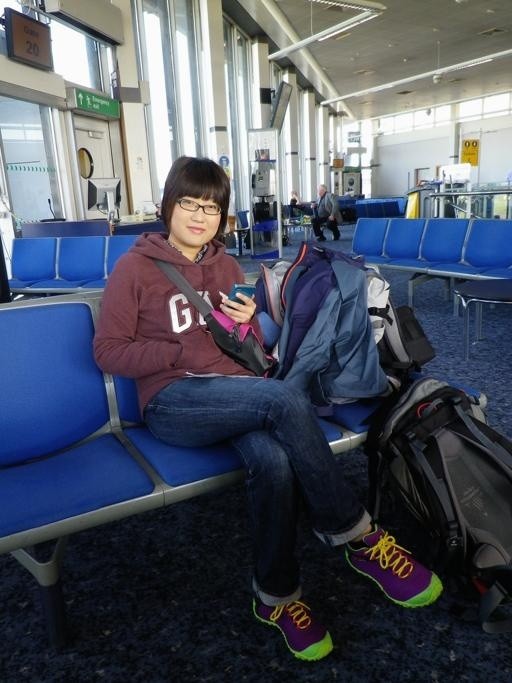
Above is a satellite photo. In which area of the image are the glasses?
[175,198,225,215]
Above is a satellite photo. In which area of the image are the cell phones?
[230,283,257,310]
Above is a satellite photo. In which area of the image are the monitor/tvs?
[88,178,120,221]
[4,7,53,71]
[271,81,293,135]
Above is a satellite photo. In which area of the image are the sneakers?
[253,598,334,662]
[344,523,443,609]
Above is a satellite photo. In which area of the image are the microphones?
[40,199,66,222]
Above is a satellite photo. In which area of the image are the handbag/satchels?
[205,311,277,377]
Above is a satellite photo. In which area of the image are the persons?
[310,185,342,242]
[289,190,300,205]
[88,152,445,663]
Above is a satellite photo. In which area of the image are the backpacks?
[364,376,512,604]
